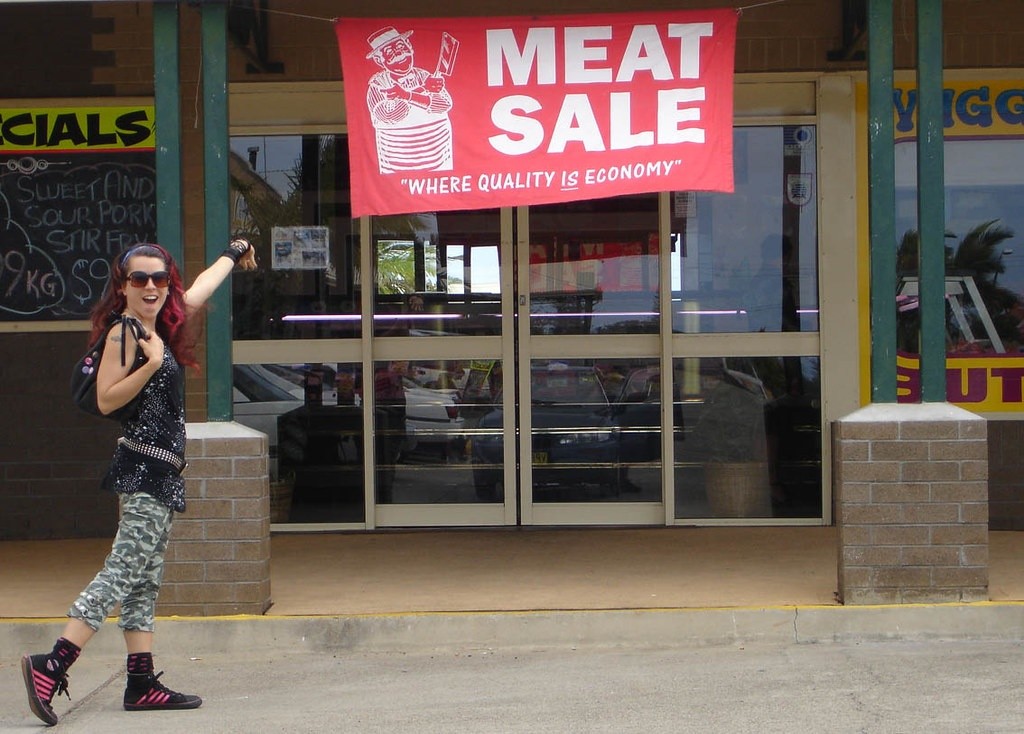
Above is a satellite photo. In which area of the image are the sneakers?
[122,673,202,711]
[20,649,72,727]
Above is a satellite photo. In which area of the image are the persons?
[357,296,424,504]
[20,238,257,726]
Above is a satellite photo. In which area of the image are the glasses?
[119,270,171,288]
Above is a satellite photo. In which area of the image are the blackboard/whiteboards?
[0,148,157,331]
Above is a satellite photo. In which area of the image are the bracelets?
[221,238,250,266]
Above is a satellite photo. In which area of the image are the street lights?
[993,249,1013,284]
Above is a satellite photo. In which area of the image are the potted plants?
[684,376,773,521]
[242,419,308,523]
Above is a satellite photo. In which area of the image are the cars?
[232,363,490,482]
[615,365,769,492]
[471,363,622,499]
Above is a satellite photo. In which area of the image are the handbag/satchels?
[69,314,150,423]
